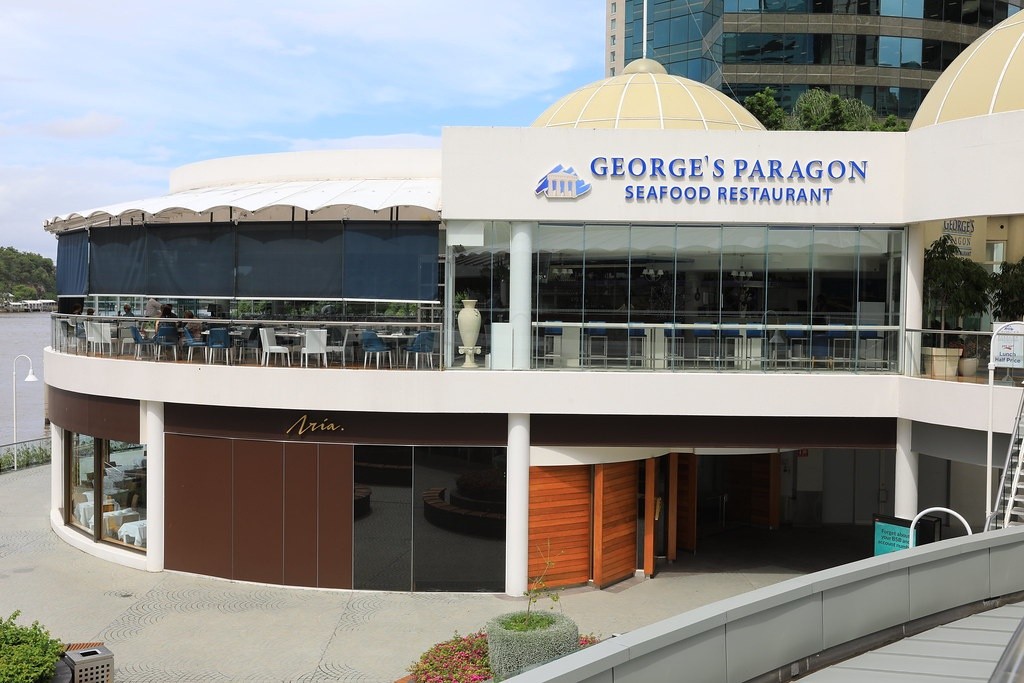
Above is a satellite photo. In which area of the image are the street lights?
[13,354,39,471]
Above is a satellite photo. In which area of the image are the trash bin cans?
[64,646,114,683]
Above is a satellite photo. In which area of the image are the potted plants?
[486,538,578,680]
[922,234,1024,381]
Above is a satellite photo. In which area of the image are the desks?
[273,333,304,366]
[200,330,244,366]
[142,329,157,360]
[376,334,419,370]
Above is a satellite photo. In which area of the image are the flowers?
[406,627,605,683]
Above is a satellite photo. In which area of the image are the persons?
[87,308,94,315]
[153,304,181,360]
[620,298,634,310]
[145,298,163,317]
[123,305,135,317]
[69,304,85,334]
[178,311,202,359]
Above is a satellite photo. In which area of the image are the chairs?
[532,321,885,370]
[59,319,437,367]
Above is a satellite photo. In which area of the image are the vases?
[457,299,481,368]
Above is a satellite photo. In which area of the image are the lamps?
[641,253,664,277]
[730,253,753,285]
[551,253,574,281]
